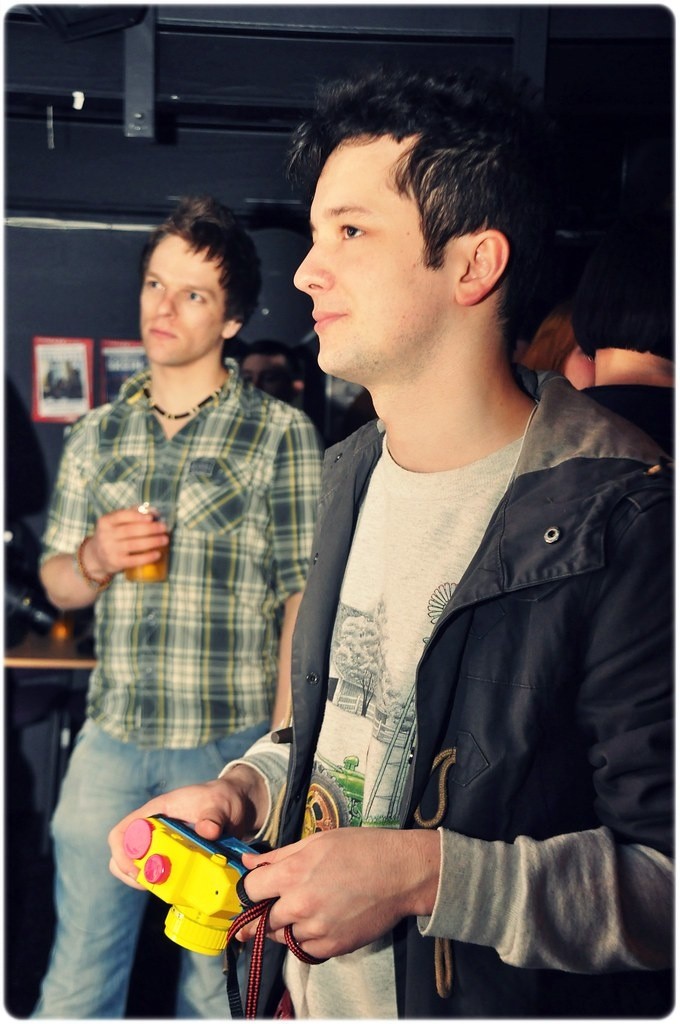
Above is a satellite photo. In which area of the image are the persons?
[31,195,321,1019]
[238,339,304,405]
[512,245,674,465]
[105,70,674,1019]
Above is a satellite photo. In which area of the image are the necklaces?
[142,376,225,420]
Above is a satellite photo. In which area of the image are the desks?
[4,638,102,672]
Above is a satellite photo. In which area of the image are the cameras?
[123,814,272,955]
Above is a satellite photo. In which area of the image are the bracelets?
[76,535,112,591]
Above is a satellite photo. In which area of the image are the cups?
[126,501,177,584]
